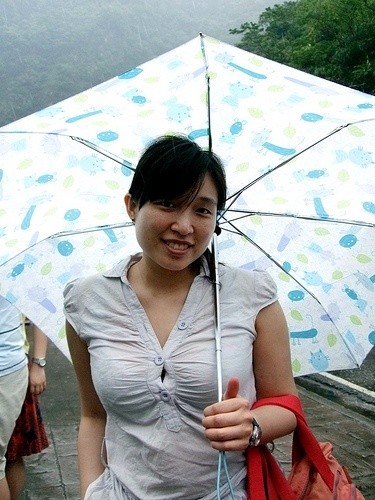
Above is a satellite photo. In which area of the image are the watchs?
[249,417,263,447]
[32,357,47,367]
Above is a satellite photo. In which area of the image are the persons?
[6,313,50,500]
[62,136,301,500]
[0,299,47,500]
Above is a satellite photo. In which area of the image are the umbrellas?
[0,32,375,500]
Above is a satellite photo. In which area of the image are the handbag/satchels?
[247,394,364,500]
